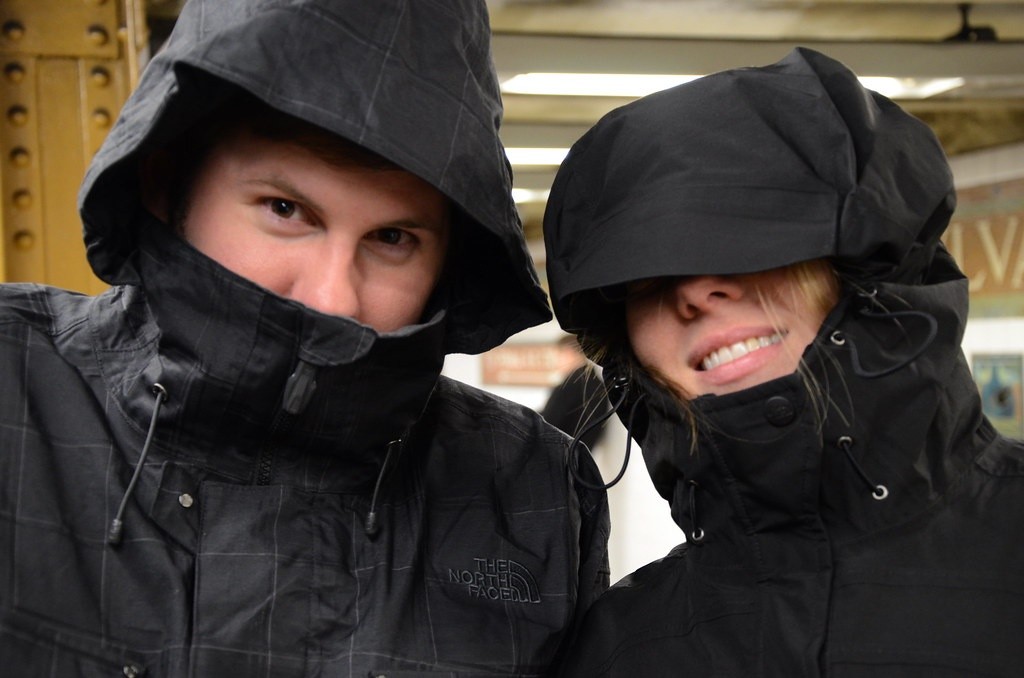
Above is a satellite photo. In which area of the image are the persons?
[0,1,610,678]
[541,47,1024,675]
